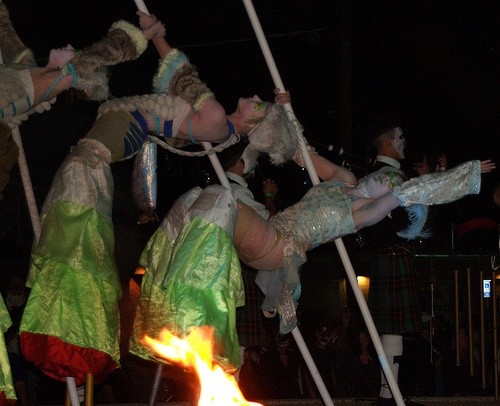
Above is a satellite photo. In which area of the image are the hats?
[82,71,109,101]
[247,104,305,165]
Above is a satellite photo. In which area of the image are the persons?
[127,85,494,380]
[19,11,298,384]
[0,0,166,201]
[360,106,450,406]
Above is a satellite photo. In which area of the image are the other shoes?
[375,395,426,406]
